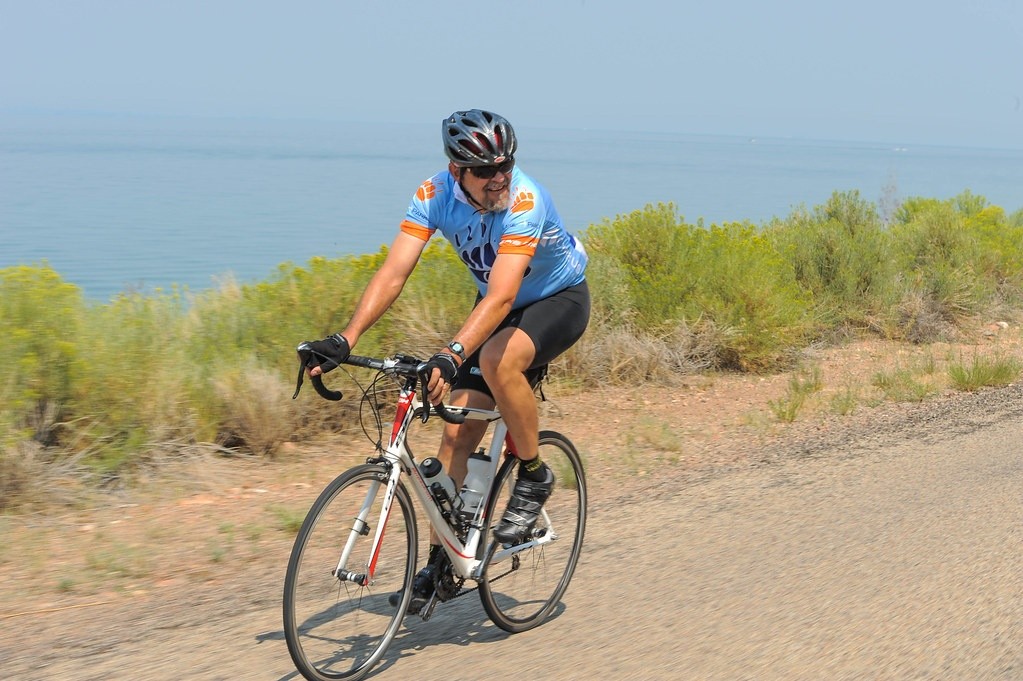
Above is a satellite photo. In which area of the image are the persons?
[302,110,592,613]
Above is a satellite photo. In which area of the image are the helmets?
[442,109,517,167]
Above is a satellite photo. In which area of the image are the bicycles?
[282,337,590,681]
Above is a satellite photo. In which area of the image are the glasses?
[467,155,515,179]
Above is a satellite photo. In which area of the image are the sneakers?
[389,562,453,613]
[493,467,555,546]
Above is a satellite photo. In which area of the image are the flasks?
[459,446,492,522]
[420,457,462,512]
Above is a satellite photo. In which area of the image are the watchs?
[444,341,468,365]
